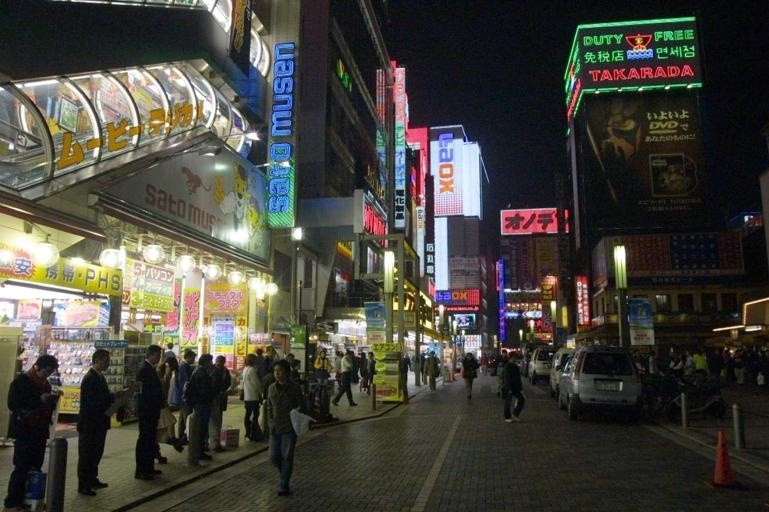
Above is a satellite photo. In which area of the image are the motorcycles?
[643,369,727,422]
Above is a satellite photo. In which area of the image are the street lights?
[383,250,394,342]
[452,320,457,370]
[550,301,557,345]
[461,329,465,355]
[438,304,444,375]
[614,244,630,347]
[519,329,523,359]
[530,320,535,342]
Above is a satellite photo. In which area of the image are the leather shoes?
[79,479,107,495]
[332,399,357,406]
[189,445,225,467]
[135,470,161,480]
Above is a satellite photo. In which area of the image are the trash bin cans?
[310,384,330,416]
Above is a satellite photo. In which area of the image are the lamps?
[21,220,278,299]
[161,129,294,169]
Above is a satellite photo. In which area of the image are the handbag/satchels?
[156,408,177,430]
[239,389,244,400]
[24,470,47,499]
[221,425,240,447]
[498,384,508,400]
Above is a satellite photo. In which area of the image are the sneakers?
[505,413,520,423]
[278,486,289,496]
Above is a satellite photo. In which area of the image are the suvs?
[520,345,642,420]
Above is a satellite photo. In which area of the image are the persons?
[312,347,378,406]
[459,349,526,423]
[4,355,58,508]
[632,341,769,387]
[76,349,123,495]
[134,344,166,480]
[403,350,441,392]
[154,342,303,496]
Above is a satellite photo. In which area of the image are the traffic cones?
[309,391,322,424]
[705,430,743,491]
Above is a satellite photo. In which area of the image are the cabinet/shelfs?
[0,327,162,425]
